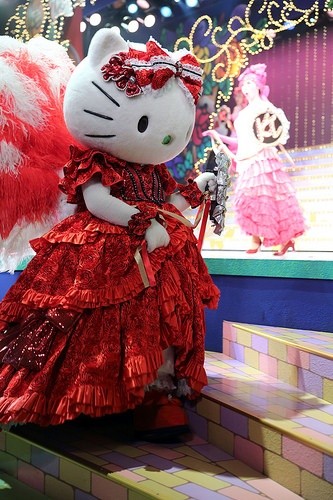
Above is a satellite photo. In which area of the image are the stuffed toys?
[0,27,219,442]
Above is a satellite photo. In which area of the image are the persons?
[202,62,305,256]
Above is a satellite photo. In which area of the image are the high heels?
[275,241,295,256]
[247,240,262,253]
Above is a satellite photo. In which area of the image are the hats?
[237,62,269,97]
